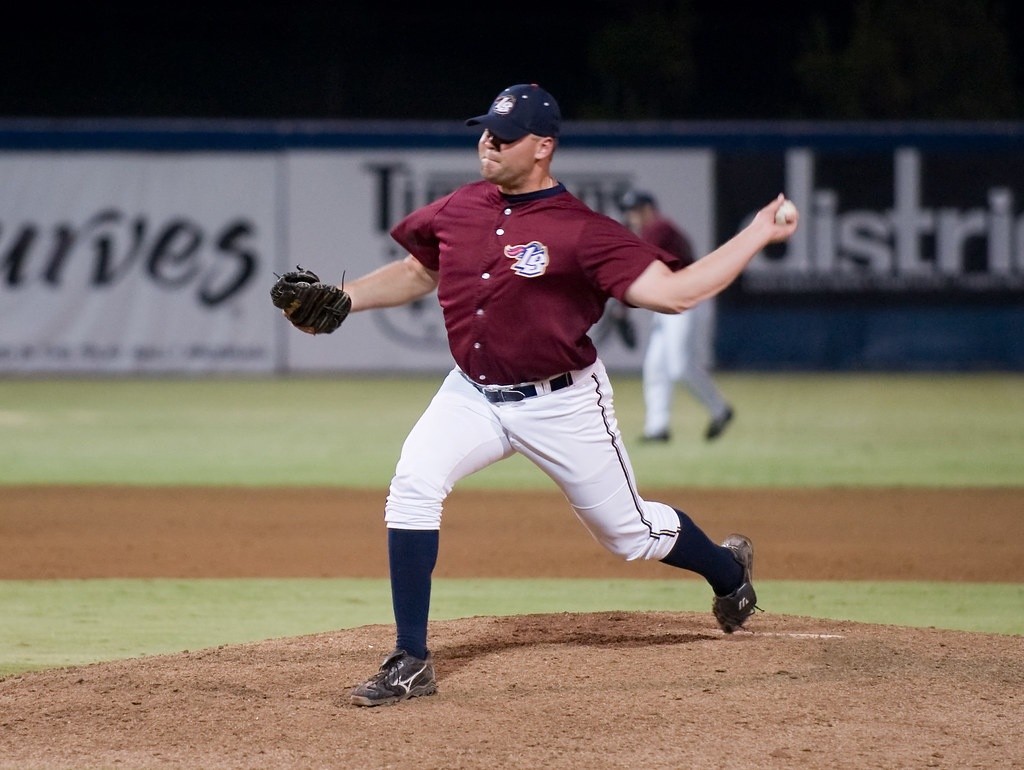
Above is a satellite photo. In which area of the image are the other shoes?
[707,408,733,437]
[640,429,670,444]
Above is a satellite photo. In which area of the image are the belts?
[460,372,574,405]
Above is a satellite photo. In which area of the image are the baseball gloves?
[269,269,352,334]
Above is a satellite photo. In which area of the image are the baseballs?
[774,199,797,225]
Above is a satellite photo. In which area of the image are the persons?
[614,188,734,441]
[284,82,798,708]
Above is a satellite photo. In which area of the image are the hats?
[618,191,654,211]
[465,84,561,142]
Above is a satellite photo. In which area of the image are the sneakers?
[712,533,765,634]
[351,649,437,707]
